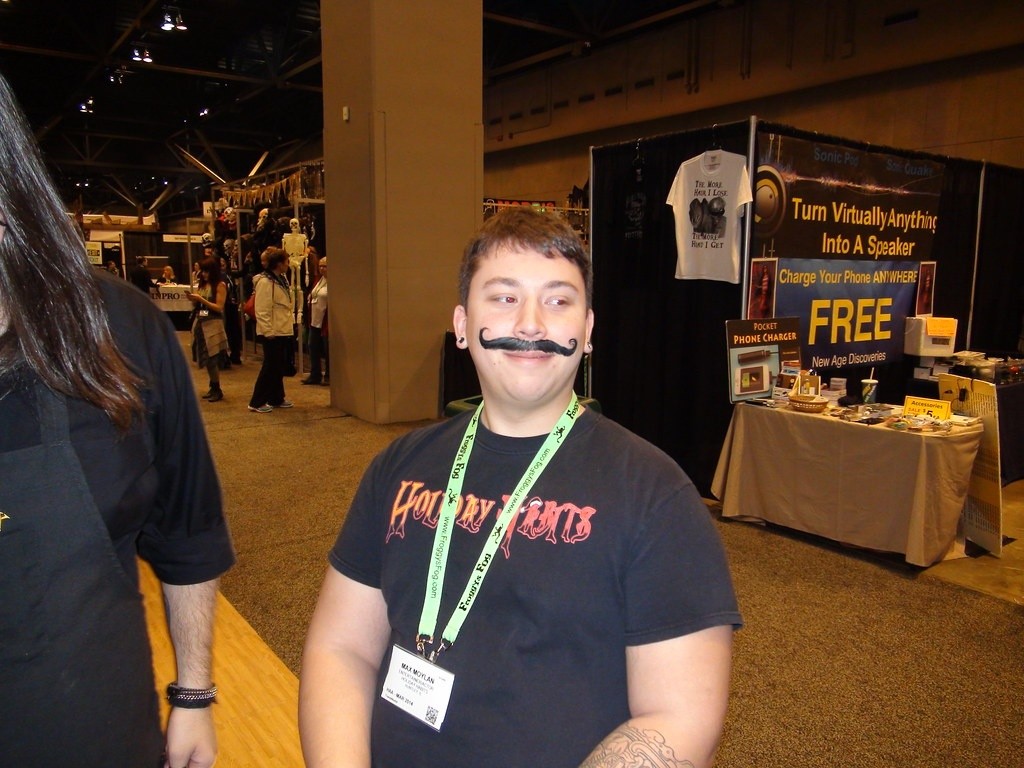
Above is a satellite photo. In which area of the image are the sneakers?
[266,401,294,407]
[248,404,273,412]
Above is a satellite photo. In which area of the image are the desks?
[710,388,985,579]
[909,375,1024,496]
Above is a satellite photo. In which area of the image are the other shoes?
[301,377,321,384]
[321,381,330,385]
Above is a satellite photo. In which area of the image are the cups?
[861,378,878,404]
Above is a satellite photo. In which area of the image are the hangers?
[703,123,724,168]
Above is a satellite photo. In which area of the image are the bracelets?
[167,683,217,709]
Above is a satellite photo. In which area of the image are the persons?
[750,265,774,318]
[129,256,160,296]
[100,261,118,277]
[252,246,292,289]
[248,250,296,412]
[155,266,181,286]
[193,259,244,372]
[187,257,227,403]
[300,274,329,385]
[0,74,237,768]
[312,257,327,284]
[296,208,745,768]
[917,271,932,314]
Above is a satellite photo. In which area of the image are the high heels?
[202,393,213,398]
[208,393,223,402]
